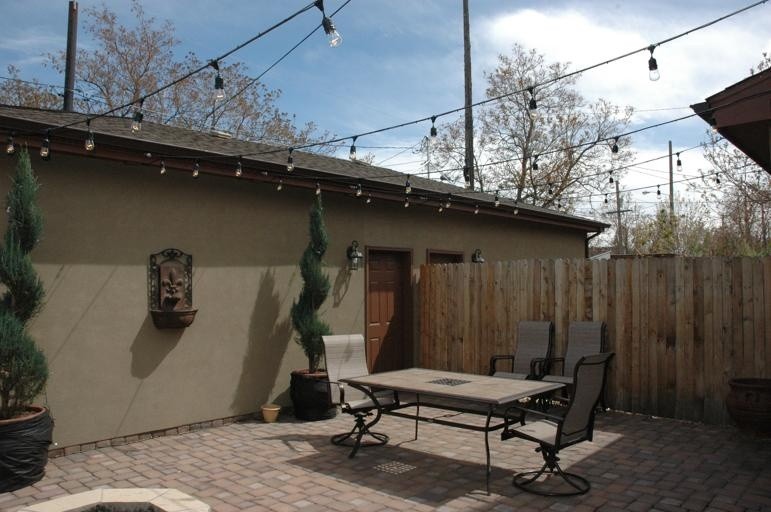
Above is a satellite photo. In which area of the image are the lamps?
[319,15,344,50]
[38,137,52,157]
[427,127,439,143]
[212,76,229,104]
[129,111,145,134]
[346,239,364,270]
[523,97,546,121]
[82,130,98,150]
[646,57,661,83]
[136,144,721,216]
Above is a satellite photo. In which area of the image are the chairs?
[501,351,617,497]
[321,334,399,445]
[488,320,552,379]
[537,320,607,412]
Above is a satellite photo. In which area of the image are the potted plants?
[289,192,337,421]
[0,145,53,494]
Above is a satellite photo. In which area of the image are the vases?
[260,403,281,423]
[726,378,771,439]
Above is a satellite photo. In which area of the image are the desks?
[336,367,567,496]
